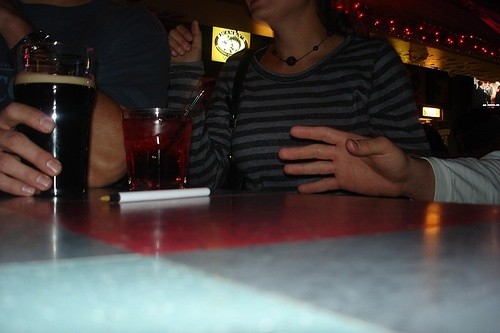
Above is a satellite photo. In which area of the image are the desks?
[0,189,500,333]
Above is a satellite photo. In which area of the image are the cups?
[123,105,195,196]
[14,32,97,199]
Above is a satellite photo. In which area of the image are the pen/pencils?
[99,187,211,203]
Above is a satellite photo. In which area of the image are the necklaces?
[272,30,334,66]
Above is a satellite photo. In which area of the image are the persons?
[0,0,170,197]
[278,125,500,204]
[167,0,452,193]
